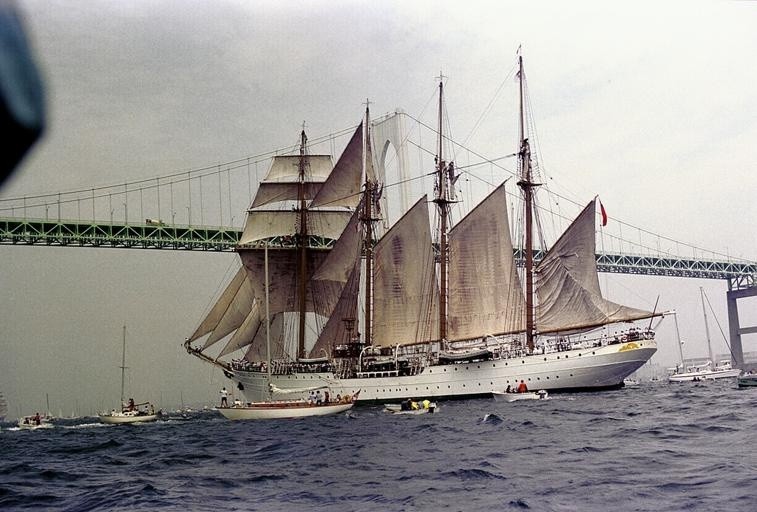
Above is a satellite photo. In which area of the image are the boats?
[489,390,552,404]
[623,375,641,386]
[95,325,160,423]
[385,402,440,417]
[736,371,757,389]
[15,414,56,431]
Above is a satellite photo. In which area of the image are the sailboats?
[7,392,232,422]
[667,285,743,383]
[219,242,363,419]
[181,43,678,406]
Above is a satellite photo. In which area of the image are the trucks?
[146,218,166,226]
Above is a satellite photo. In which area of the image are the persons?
[33,412,41,426]
[230,356,332,373]
[127,398,155,416]
[517,380,529,393]
[505,383,512,394]
[406,397,430,410]
[310,389,341,406]
[510,335,588,356]
[511,388,515,392]
[593,327,652,343]
[23,417,30,424]
[219,386,229,408]
